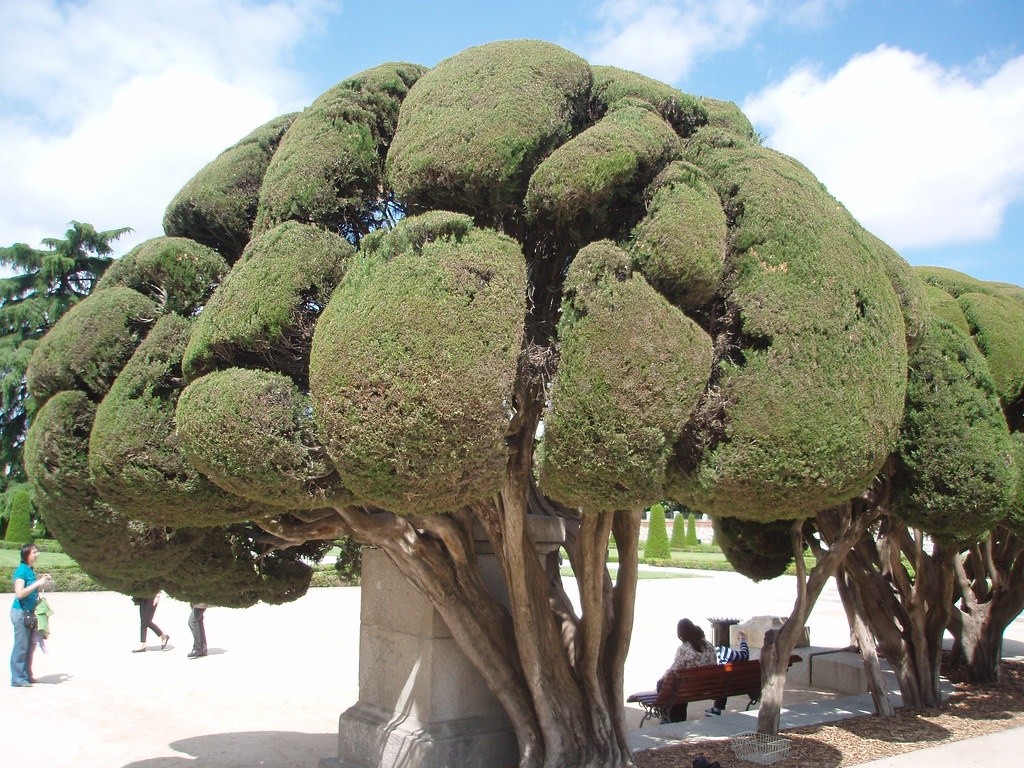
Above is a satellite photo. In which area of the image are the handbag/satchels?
[24,611,38,629]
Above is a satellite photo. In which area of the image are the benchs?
[627,653,803,727]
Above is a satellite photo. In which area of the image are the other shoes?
[161,635,169,650]
[133,647,146,652]
[28,678,38,683]
[188,650,207,658]
[12,682,33,686]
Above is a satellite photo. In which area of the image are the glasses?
[31,551,41,556]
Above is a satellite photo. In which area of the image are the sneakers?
[704,707,721,717]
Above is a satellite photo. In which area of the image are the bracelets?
[737,636,745,639]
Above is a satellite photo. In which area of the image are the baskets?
[729,731,793,765]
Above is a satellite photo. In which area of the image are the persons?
[694,625,749,716]
[132,592,169,653]
[188,603,208,659]
[10,544,51,686]
[660,618,717,724]
